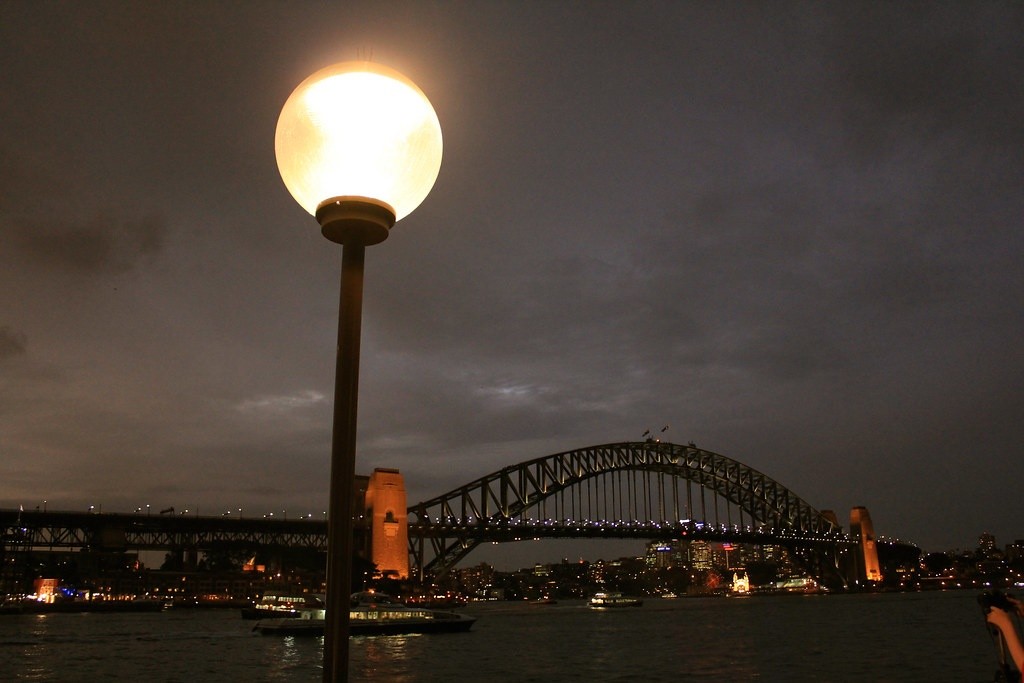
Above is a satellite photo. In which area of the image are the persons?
[987,597,1024,683]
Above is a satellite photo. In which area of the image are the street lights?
[239,508,243,521]
[147,503,150,517]
[43,499,47,513]
[273,60,444,683]
[283,509,286,522]
[323,510,327,522]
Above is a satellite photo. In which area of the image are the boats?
[590,590,643,607]
[252,590,479,637]
[240,609,301,621]
[529,598,558,605]
[662,593,677,598]
[455,600,468,607]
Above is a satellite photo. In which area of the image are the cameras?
[978,589,1014,626]
